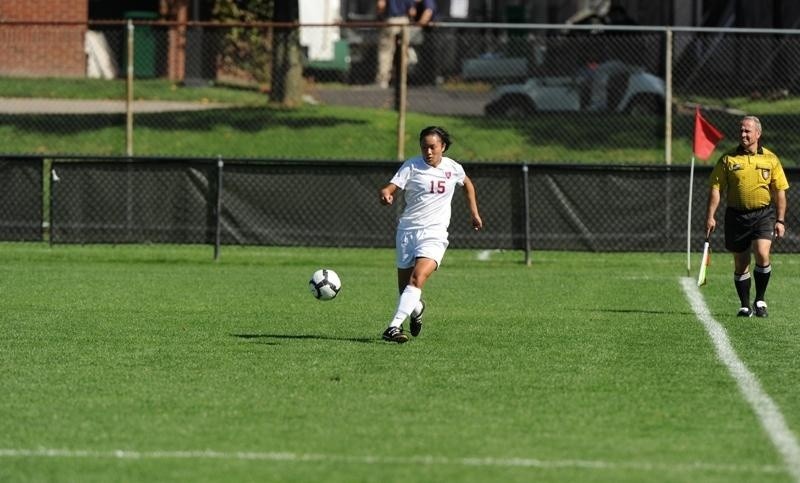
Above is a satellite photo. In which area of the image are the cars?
[484,48,679,118]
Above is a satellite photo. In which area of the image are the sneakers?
[382,327,409,343]
[410,299,426,337]
[737,300,769,318]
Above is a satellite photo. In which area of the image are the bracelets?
[777,218,786,227]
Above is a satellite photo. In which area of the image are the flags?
[692,106,725,163]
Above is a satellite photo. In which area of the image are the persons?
[414,0,442,85]
[377,125,483,345]
[375,1,419,89]
[704,115,791,319]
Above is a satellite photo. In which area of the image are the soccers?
[308,269,342,301]
